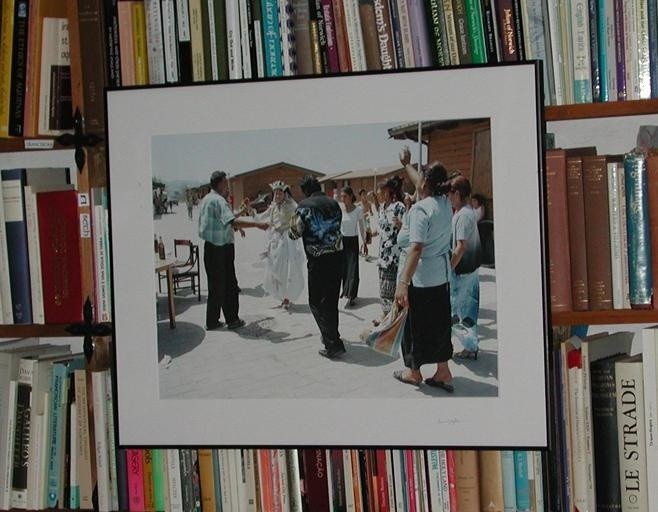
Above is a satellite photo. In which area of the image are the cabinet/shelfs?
[1,98,658,512]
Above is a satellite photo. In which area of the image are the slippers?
[424,376,455,393]
[393,369,422,387]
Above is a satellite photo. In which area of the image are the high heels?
[455,345,479,360]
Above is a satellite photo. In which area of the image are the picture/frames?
[102,57,553,452]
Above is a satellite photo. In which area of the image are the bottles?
[153,233,158,252]
[158,236,165,260]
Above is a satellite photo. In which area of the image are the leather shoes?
[318,343,346,359]
[227,320,244,329]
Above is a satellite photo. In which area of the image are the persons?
[182,143,491,391]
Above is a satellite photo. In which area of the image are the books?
[0,447,544,510]
[0,0,657,137]
[0,138,121,447]
[538,126,658,511]
